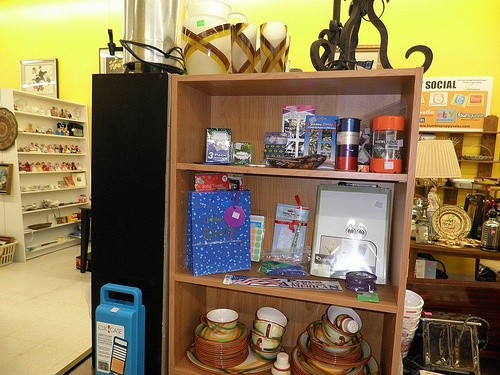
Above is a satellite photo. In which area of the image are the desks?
[78,205,91,273]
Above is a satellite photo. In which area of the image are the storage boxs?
[0,238,19,268]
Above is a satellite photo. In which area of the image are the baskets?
[0,240,19,267]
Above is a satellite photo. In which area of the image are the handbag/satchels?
[187,186,251,276]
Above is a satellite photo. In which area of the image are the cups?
[400,290,424,356]
[230,22,290,73]
[200,308,239,334]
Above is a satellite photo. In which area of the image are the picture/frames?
[334,45,381,69]
[0,164,13,195]
[0,236,14,246]
[20,58,59,99]
[309,184,391,286]
[99,48,125,74]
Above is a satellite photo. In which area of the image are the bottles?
[334,314,358,335]
[78,194,87,203]
[270,352,292,375]
[479,212,500,252]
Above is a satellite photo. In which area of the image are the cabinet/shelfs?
[0,88,91,262]
[406,115,500,359]
[167,66,423,375]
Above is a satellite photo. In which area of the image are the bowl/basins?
[307,305,363,353]
[248,306,289,358]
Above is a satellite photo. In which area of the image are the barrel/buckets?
[123,0,184,72]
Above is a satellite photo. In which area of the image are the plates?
[432,206,472,240]
[288,331,381,375]
[185,321,287,375]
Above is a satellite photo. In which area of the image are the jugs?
[180,1,247,75]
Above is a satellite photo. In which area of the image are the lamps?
[415,140,462,243]
